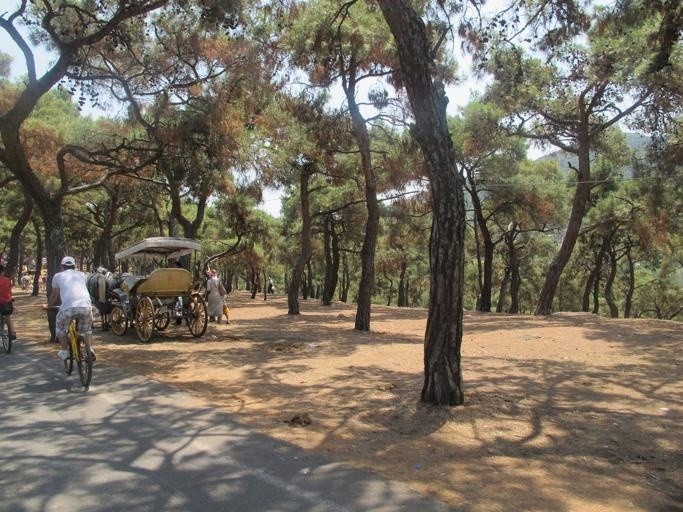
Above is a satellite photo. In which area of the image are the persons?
[0,264,15,340]
[205,267,225,324]
[218,277,230,324]
[267,277,274,293]
[42,255,97,362]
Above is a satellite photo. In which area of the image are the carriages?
[87,237,208,343]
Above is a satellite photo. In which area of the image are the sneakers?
[57,350,70,361]
[87,349,96,361]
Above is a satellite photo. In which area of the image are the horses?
[85,264,121,332]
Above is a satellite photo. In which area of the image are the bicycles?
[1,307,12,353]
[44,306,92,387]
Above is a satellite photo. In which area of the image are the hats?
[61,256,75,266]
[206,269,219,275]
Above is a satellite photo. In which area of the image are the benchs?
[136,268,193,297]
